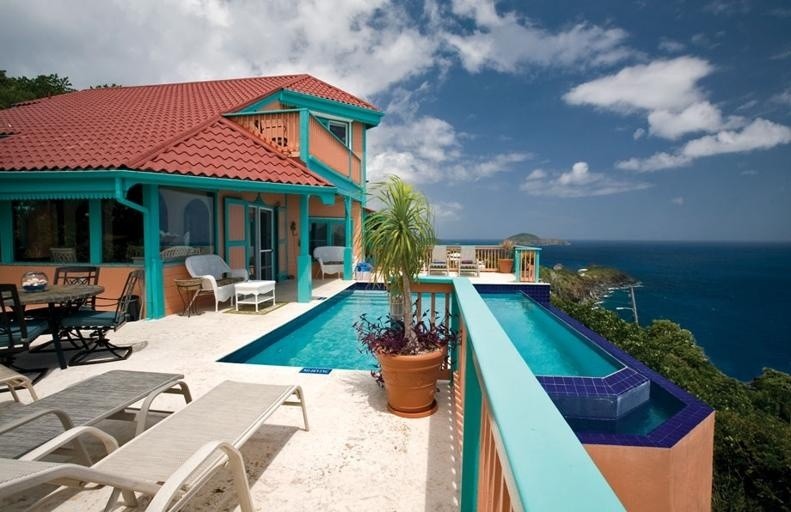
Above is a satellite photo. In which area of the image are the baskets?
[21,271,48,293]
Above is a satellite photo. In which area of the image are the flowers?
[498,239,514,258]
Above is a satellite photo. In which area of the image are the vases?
[498,258,514,273]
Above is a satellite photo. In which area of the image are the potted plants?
[353,174,464,420]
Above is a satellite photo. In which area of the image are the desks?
[173,278,204,317]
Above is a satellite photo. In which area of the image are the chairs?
[428,245,480,278]
[1,265,140,393]
[50,247,78,262]
[1,364,309,511]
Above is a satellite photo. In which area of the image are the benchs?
[313,246,358,281]
[185,252,249,313]
[130,245,200,264]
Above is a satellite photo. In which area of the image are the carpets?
[224,302,289,314]
